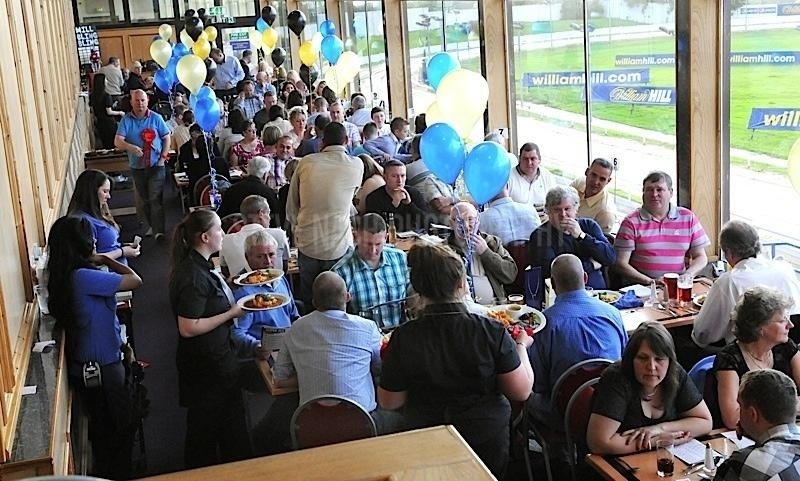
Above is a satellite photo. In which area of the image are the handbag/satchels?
[83,359,102,392]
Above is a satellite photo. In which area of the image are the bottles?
[702,443,715,469]
[282,239,291,259]
[388,213,397,244]
[649,279,660,304]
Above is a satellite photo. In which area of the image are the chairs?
[192,173,229,205]
[697,368,723,430]
[289,396,378,450]
[688,354,716,394]
[200,184,223,206]
[503,239,529,294]
[564,377,601,480]
[523,360,613,481]
[220,213,243,235]
[604,233,615,273]
[227,220,244,234]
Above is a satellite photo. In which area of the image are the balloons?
[148,8,221,135]
[420,53,511,205]
[248,6,361,94]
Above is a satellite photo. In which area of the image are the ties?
[208,268,240,329]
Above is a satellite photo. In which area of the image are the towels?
[611,289,644,309]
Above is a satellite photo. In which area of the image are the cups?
[508,294,525,304]
[677,274,694,308]
[656,439,674,476]
[663,273,678,307]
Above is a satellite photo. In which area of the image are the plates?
[236,293,292,311]
[483,304,547,340]
[586,289,623,303]
[233,269,285,286]
[692,294,709,307]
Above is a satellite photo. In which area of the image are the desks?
[83,152,144,226]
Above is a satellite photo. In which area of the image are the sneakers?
[154,233,164,242]
[145,227,154,237]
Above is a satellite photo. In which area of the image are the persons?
[379,127,800,481]
[44,47,250,481]
[210,48,427,431]
